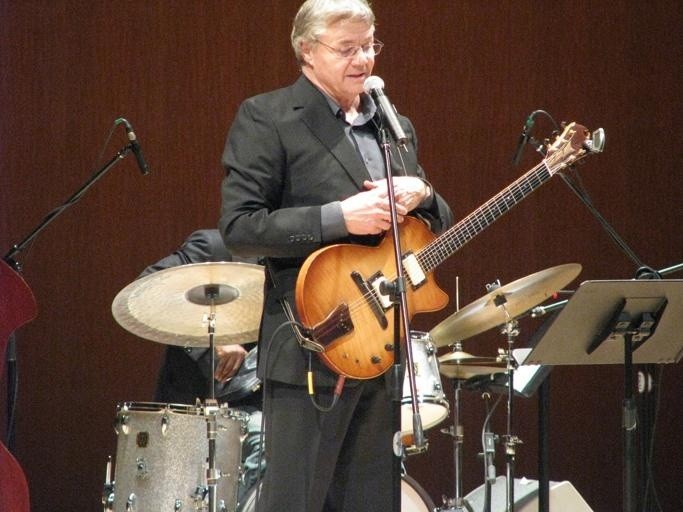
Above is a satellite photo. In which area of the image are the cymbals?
[438,350,510,379]
[110,263,266,347]
[428,262,583,350]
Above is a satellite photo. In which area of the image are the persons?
[131,221,270,511]
[222,0,455,511]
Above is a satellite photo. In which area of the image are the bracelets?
[423,184,430,200]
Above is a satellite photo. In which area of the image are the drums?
[111,400,249,512]
[400,330,451,436]
[239,472,435,512]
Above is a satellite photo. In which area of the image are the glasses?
[311,36,384,59]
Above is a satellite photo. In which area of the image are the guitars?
[295,122,595,381]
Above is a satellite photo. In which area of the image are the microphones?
[512,119,534,166]
[125,124,150,176]
[363,76,408,147]
